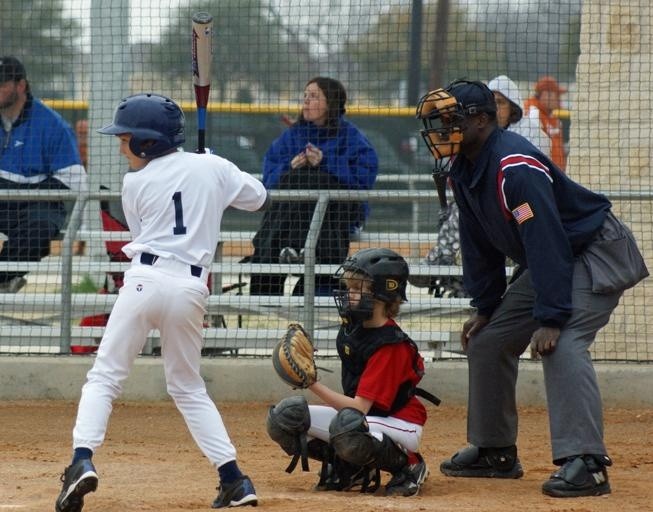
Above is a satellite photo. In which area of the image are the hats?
[532,77,567,93]
[0,57,26,81]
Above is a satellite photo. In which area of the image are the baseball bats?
[192,12,214,154]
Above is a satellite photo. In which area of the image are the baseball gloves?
[272,320,321,390]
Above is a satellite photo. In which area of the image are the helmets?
[416,78,498,172]
[333,248,408,326]
[96,94,186,159]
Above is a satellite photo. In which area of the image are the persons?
[266,248,441,499]
[55,93,268,512]
[416,78,649,497]
[522,75,568,173]
[487,74,554,160]
[0,56,88,293]
[250,77,379,296]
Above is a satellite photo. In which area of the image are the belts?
[141,252,202,277]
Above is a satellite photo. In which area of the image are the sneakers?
[279,247,304,264]
[325,468,377,489]
[410,261,451,287]
[55,459,98,512]
[543,454,612,496]
[440,446,523,478]
[384,452,429,496]
[213,476,258,508]
[1,277,26,292]
